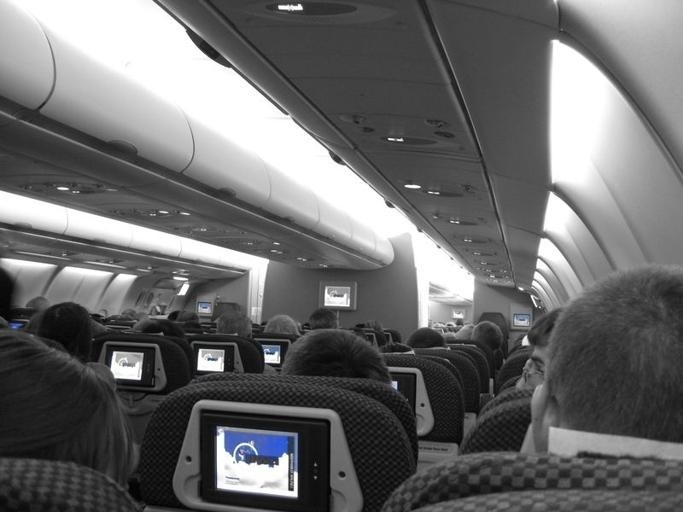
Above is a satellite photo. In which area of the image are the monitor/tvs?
[513,313,531,326]
[388,373,415,414]
[8,320,27,328]
[201,413,331,511]
[259,341,287,366]
[193,344,234,376]
[196,301,212,314]
[319,280,357,311]
[105,345,155,388]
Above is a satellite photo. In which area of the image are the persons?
[513,304,570,397]
[519,262,683,462]
[1,268,505,510]
